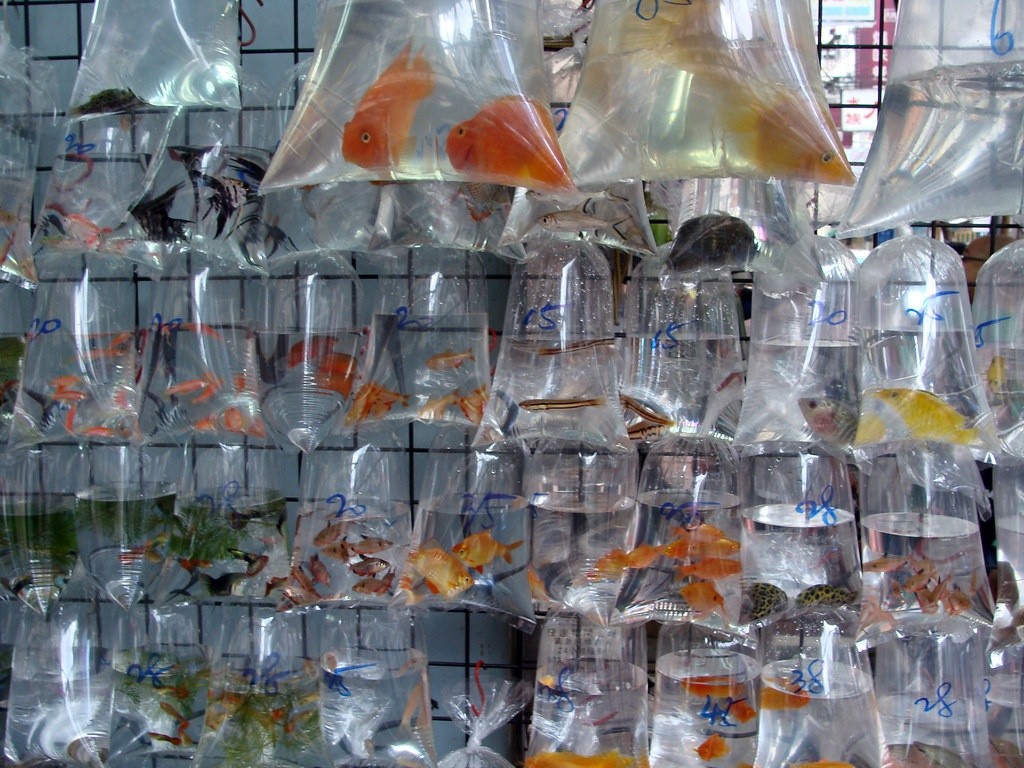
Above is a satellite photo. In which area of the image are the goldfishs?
[0,0,1023,767]
